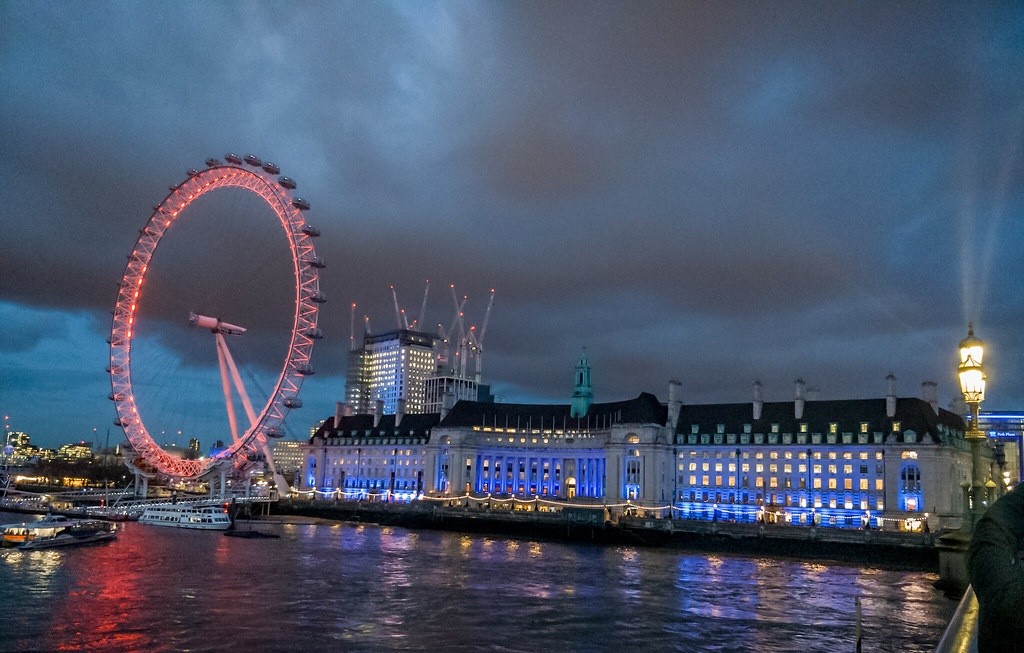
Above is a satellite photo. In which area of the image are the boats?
[0,505,119,551]
[136,502,231,530]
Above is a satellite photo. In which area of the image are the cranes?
[349,279,495,384]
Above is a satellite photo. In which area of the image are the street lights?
[924,512,931,532]
[488,492,492,508]
[466,491,469,506]
[958,323,996,541]
[386,489,389,503]
[627,499,631,516]
[812,507,816,525]
[668,497,673,518]
[714,504,718,521]
[336,488,339,501]
[865,510,871,529]
[361,489,363,502]
[312,486,316,499]
[534,495,539,511]
[761,506,766,524]
[291,486,294,498]
[511,493,515,509]
